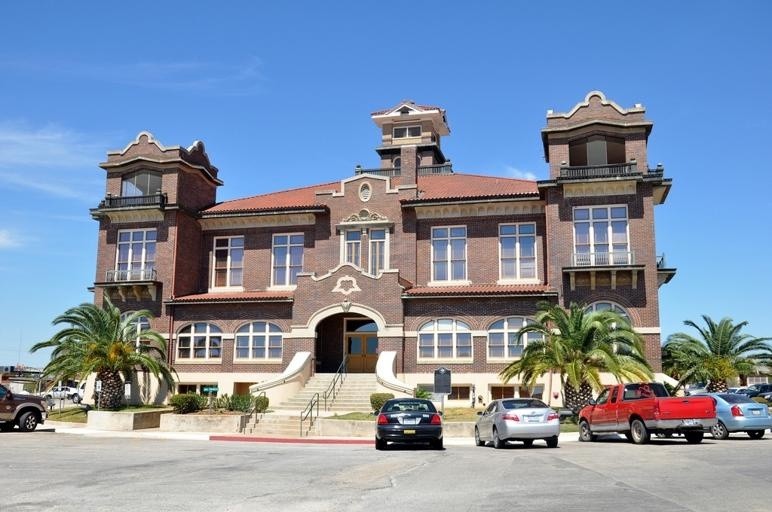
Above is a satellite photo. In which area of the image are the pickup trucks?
[572,381,717,448]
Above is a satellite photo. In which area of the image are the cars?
[372,397,444,450]
[677,381,772,406]
[472,398,562,450]
[38,385,75,400]
[682,392,771,440]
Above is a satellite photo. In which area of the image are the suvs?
[0,382,49,432]
[68,381,87,404]
